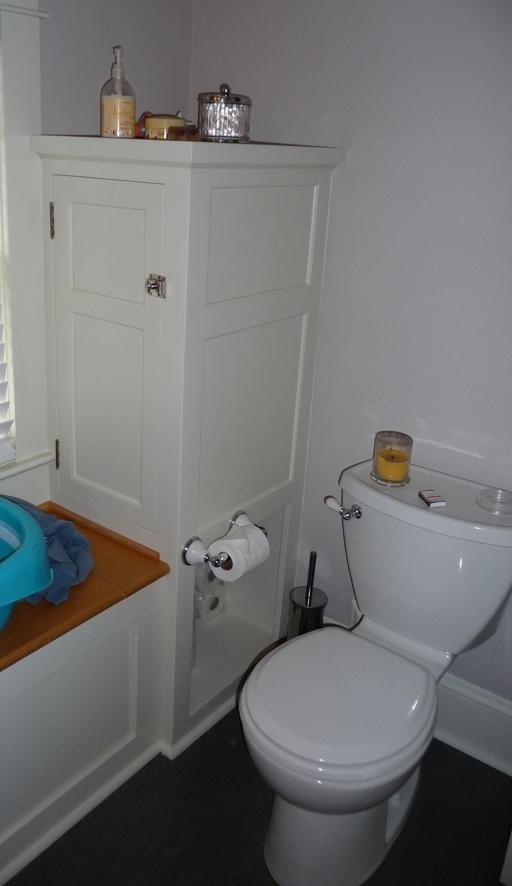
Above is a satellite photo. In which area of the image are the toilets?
[239,460,511,885]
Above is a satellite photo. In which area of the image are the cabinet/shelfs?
[49,176,332,529]
